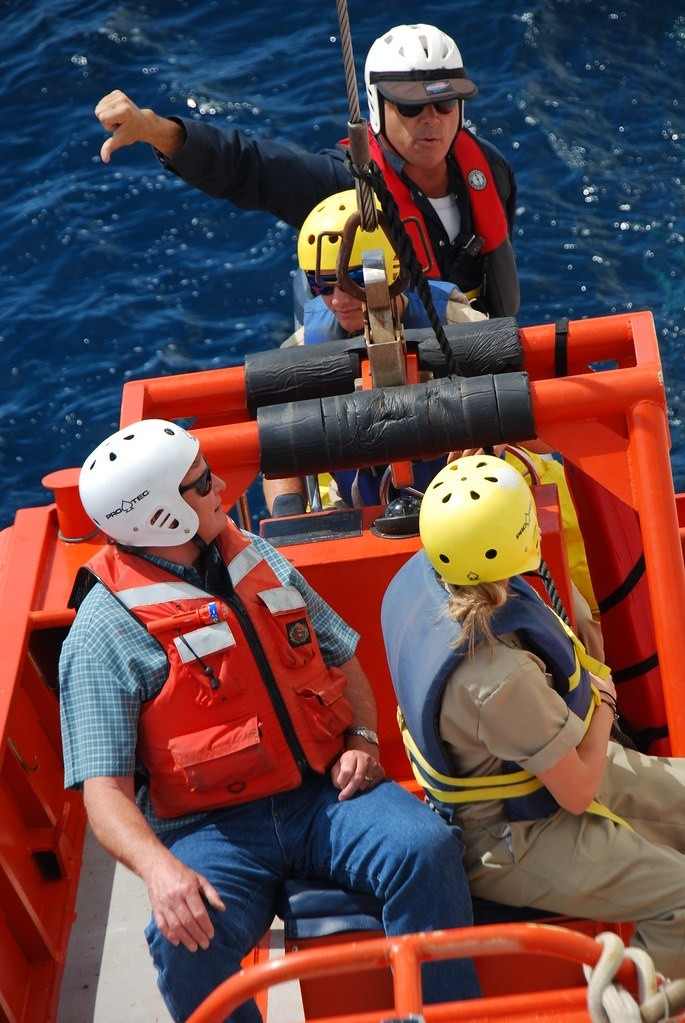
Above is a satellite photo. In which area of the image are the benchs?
[274,879,594,1022]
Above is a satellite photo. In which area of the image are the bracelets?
[598,690,619,714]
[600,698,619,721]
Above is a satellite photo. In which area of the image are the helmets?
[298,189,401,288]
[365,23,479,134]
[417,454,541,585]
[78,419,200,547]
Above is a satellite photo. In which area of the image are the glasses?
[178,457,212,499]
[306,268,364,296]
[382,96,456,117]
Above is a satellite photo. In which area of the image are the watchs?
[351,725,380,747]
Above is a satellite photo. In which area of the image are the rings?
[364,776,373,782]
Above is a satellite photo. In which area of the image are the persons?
[55,417,480,1023]
[263,190,491,519]
[381,453,685,993]
[94,20,521,325]
